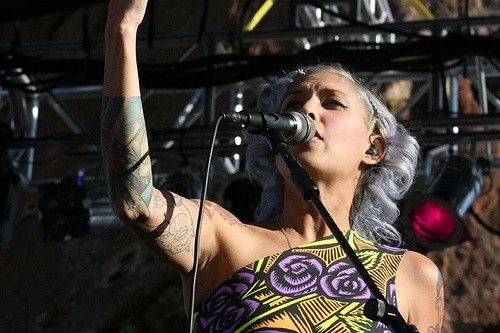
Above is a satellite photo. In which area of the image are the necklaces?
[275,213,293,249]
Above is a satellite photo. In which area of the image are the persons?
[102,0,444,333]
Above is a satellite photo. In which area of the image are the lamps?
[161,155,215,206]
[398,139,486,255]
[37,181,93,245]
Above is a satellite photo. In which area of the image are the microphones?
[222,110,316,144]
